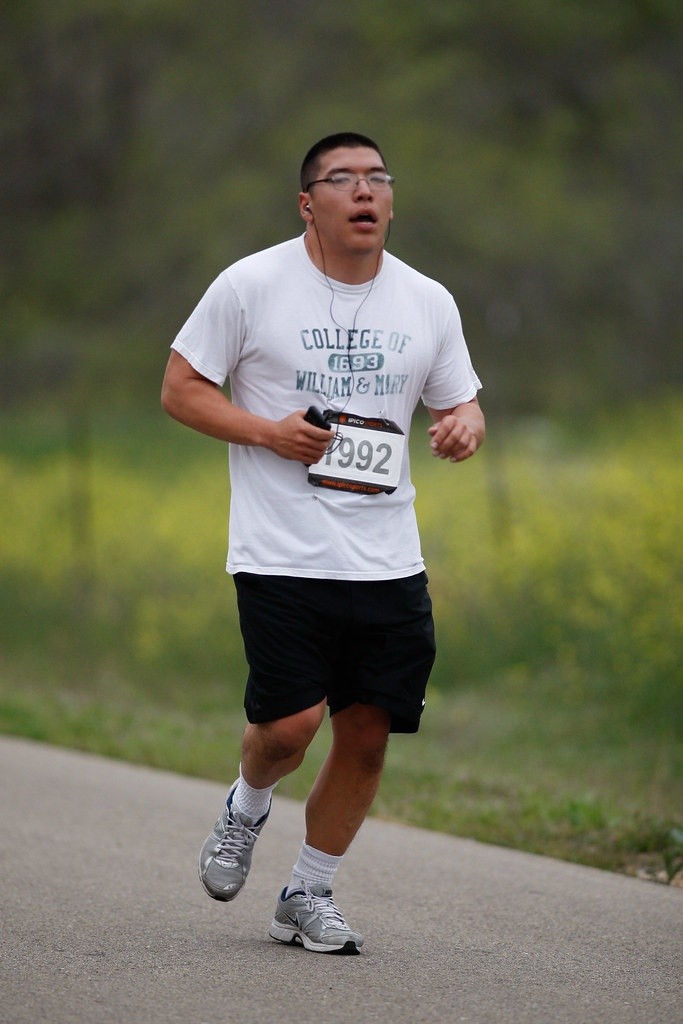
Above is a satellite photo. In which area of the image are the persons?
[157,130,489,957]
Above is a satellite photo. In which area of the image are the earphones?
[305,205,312,214]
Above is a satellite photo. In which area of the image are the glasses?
[306,171,395,194]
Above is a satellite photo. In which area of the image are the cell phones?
[301,406,331,466]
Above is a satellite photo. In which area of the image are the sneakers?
[269,884,365,956]
[199,778,271,903]
